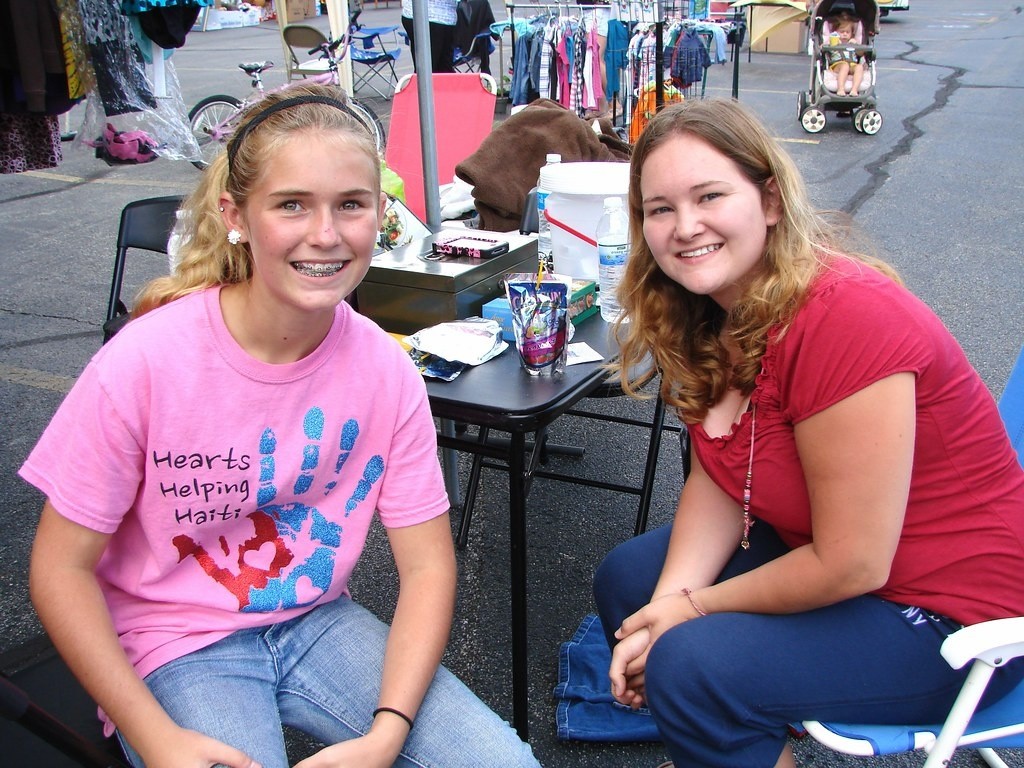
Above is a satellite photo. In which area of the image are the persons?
[401,0,458,72]
[18,86,541,768]
[818,14,863,96]
[593,98,1024,768]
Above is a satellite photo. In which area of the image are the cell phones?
[432,235,510,260]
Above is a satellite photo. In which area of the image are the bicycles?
[183,8,386,171]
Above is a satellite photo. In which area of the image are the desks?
[399,281,671,747]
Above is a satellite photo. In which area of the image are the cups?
[829,31,840,46]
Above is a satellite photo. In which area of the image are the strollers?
[796,0,883,135]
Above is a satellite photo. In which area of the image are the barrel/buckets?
[539,160,631,283]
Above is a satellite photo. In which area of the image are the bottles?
[536,152,562,274]
[166,209,196,278]
[596,195,630,324]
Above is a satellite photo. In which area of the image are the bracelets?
[373,708,413,730]
[682,589,706,616]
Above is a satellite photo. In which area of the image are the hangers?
[523,4,693,34]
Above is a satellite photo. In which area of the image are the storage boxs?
[482,280,597,341]
[276,0,316,23]
[356,229,539,334]
[751,21,806,54]
[243,12,259,26]
[219,11,243,27]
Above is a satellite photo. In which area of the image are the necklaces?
[740,406,754,550]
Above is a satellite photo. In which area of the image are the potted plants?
[494,76,511,113]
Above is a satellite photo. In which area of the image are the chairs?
[101,196,187,349]
[283,25,336,83]
[384,72,497,222]
[348,17,402,102]
[802,340,1024,768]
[453,29,495,74]
[0,632,132,768]
